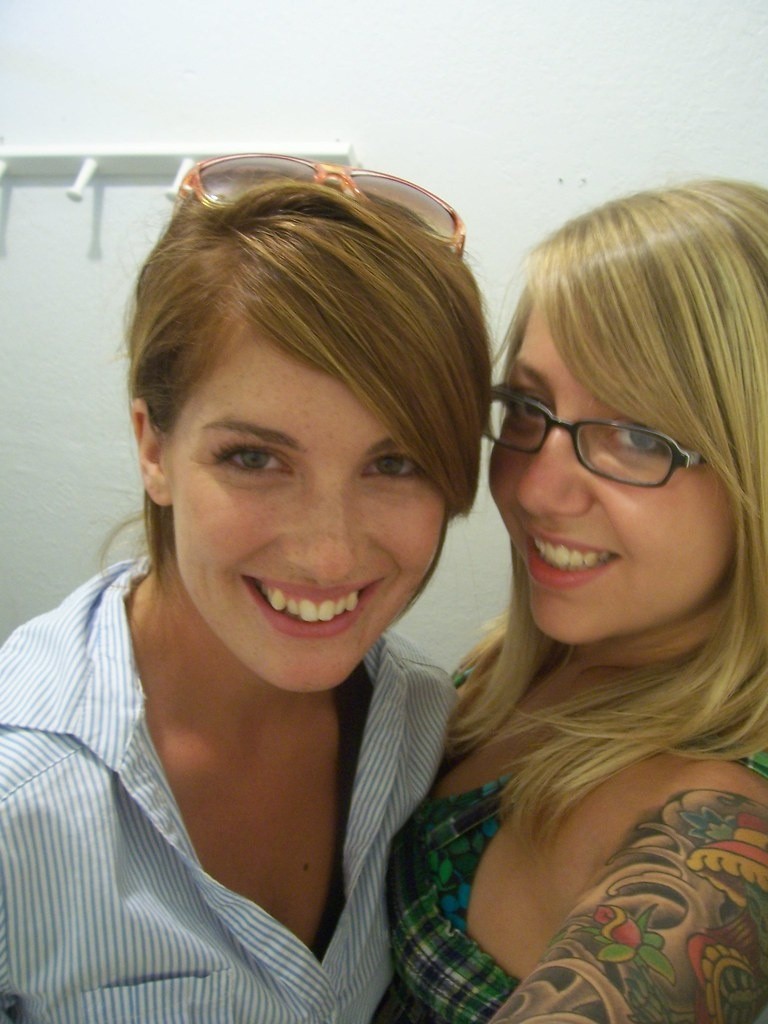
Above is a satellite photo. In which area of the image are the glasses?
[171,152,467,263]
[480,382,708,488]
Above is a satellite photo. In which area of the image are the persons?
[370,179,768,1024]
[0,151,494,1024]
